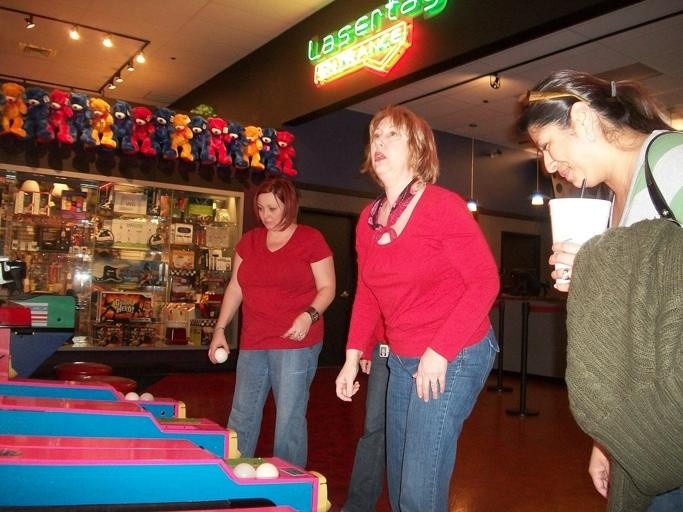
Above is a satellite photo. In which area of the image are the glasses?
[518,88,591,106]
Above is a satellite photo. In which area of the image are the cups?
[546,197,613,289]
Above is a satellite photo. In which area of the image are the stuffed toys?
[0,78,298,179]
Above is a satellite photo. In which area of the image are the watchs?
[304,305,319,321]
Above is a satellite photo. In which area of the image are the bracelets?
[215,326,224,331]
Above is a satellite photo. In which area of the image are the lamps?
[532,148,544,205]
[467,137,477,212]
[489,73,501,89]
[0,6,152,94]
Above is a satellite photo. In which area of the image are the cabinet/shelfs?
[0,163,244,351]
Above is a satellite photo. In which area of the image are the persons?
[340,313,390,511]
[335,104,503,511]
[207,177,336,467]
[513,70,683,511]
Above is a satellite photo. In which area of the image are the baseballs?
[125,392,139,401]
[234,463,255,478]
[256,463,279,478]
[139,393,154,401]
[215,348,228,363]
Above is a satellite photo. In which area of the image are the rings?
[300,333,304,337]
